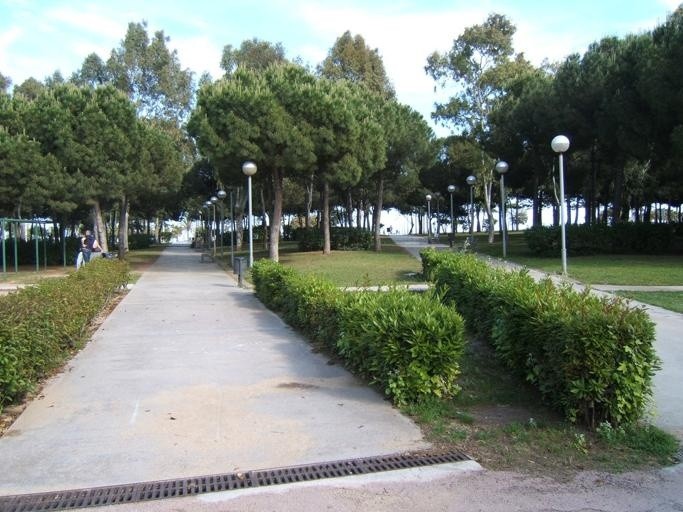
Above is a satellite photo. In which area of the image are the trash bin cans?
[101,252,118,259]
[234,257,247,277]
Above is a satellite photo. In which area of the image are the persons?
[80,229,95,265]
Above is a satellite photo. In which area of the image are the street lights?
[550,134,571,274]
[217,190,226,260]
[447,185,456,239]
[202,196,218,256]
[425,194,431,237]
[495,161,509,258]
[241,161,257,269]
[465,175,476,243]
